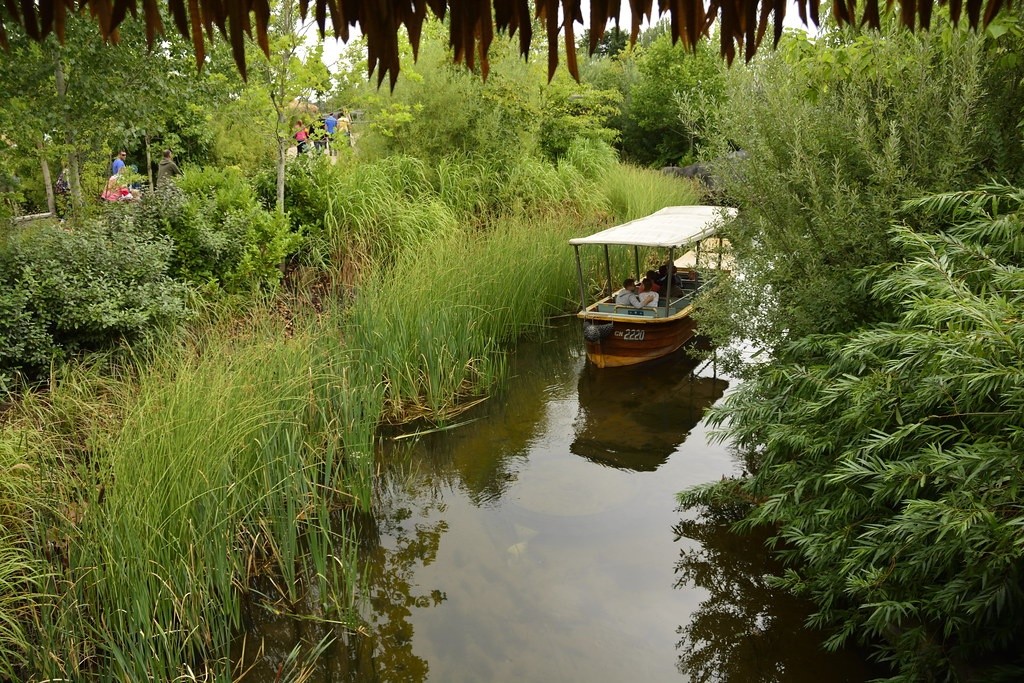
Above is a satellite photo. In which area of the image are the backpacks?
[54,172,70,194]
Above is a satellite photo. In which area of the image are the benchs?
[675,272,706,293]
[598,302,675,319]
[634,296,692,309]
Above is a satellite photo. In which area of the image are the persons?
[102,149,142,204]
[616,260,683,309]
[156,149,183,184]
[61,158,71,223]
[294,112,352,158]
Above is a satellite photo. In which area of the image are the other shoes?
[330,153,332,156]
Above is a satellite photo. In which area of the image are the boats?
[568,205,753,368]
[570,334,729,471]
